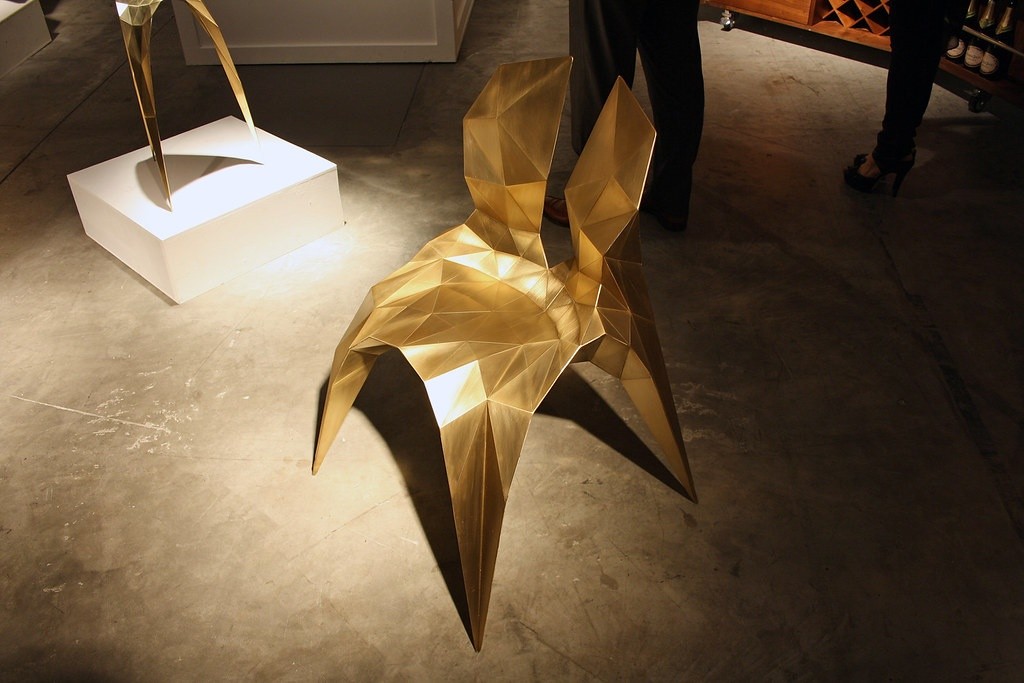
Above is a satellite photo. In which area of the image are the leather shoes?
[640,190,687,232]
[543,197,570,228]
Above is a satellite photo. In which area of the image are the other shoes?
[969,88,992,113]
[946,39,999,75]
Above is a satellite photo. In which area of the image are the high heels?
[843,148,917,198]
[965,0,1018,35]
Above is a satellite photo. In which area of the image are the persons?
[544,0,705,234]
[843,0,973,198]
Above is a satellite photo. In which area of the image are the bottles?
[944,0,1019,80]
